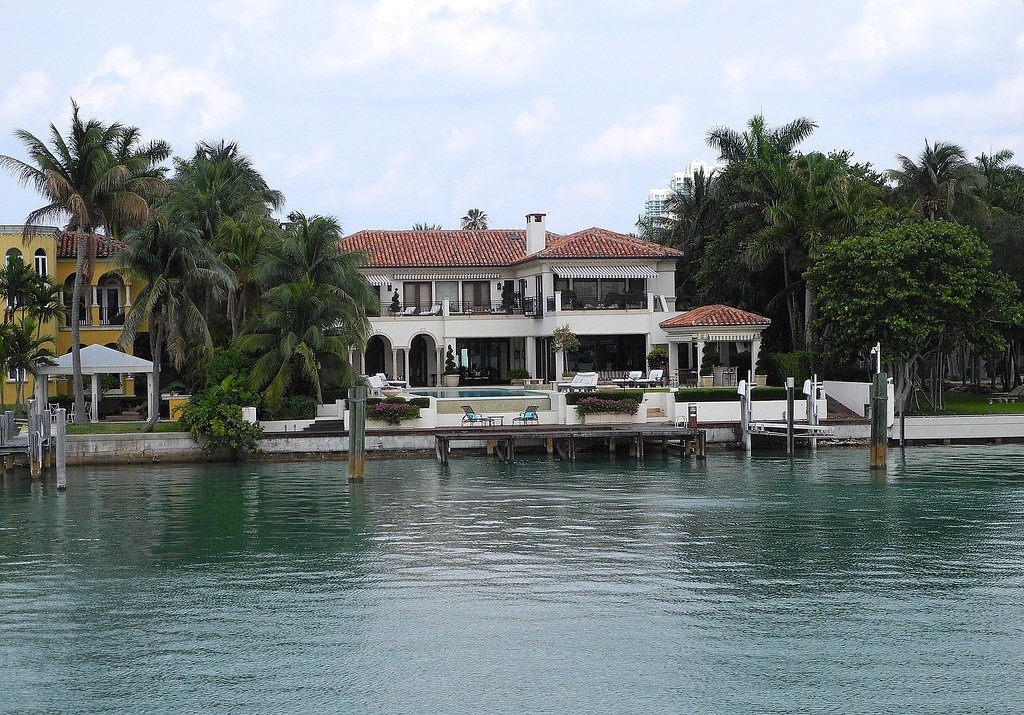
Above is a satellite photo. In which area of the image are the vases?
[581,411,635,424]
[381,390,400,398]
[367,418,421,429]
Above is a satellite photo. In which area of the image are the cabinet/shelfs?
[712,366,739,387]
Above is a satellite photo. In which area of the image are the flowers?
[380,386,401,391]
[574,397,639,420]
[365,402,422,426]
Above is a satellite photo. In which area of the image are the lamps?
[514,349,519,361]
[518,279,527,288]
[497,282,502,290]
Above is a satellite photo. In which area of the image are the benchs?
[98,398,148,421]
[523,378,544,385]
[987,397,1018,405]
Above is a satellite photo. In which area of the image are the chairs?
[48,403,60,424]
[368,373,390,397]
[418,304,441,316]
[686,371,698,388]
[611,370,666,388]
[459,406,495,428]
[394,306,416,317]
[66,402,77,424]
[460,368,490,386]
[84,401,91,422]
[511,406,540,425]
[558,372,598,392]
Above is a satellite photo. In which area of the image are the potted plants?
[755,338,770,388]
[699,341,716,387]
[560,289,645,312]
[389,288,402,317]
[501,286,515,316]
[562,372,575,382]
[441,344,460,386]
[506,368,531,386]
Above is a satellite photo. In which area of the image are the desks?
[487,416,504,427]
[722,371,737,387]
[991,392,1008,403]
